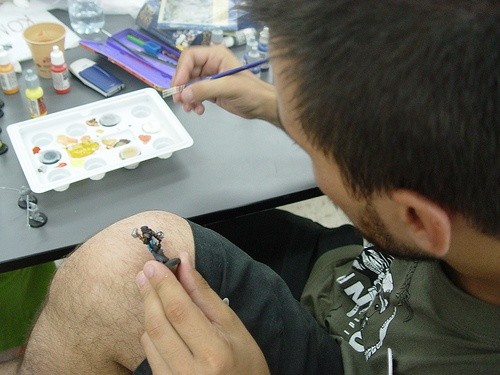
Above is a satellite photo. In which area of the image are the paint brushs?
[162,57,269,98]
[100,28,149,62]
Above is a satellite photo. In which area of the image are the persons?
[18,0,500,374]
[136,225,169,263]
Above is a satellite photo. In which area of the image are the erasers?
[144,42,161,55]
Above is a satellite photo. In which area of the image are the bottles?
[67,0,105,33]
[50,45,72,94]
[209,24,270,78]
[0,51,19,95]
[24,68,47,118]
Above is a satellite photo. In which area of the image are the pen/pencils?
[127,34,145,46]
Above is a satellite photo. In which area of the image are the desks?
[0,9,327,275]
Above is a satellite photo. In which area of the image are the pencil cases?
[79,28,181,92]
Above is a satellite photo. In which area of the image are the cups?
[23,22,65,79]
